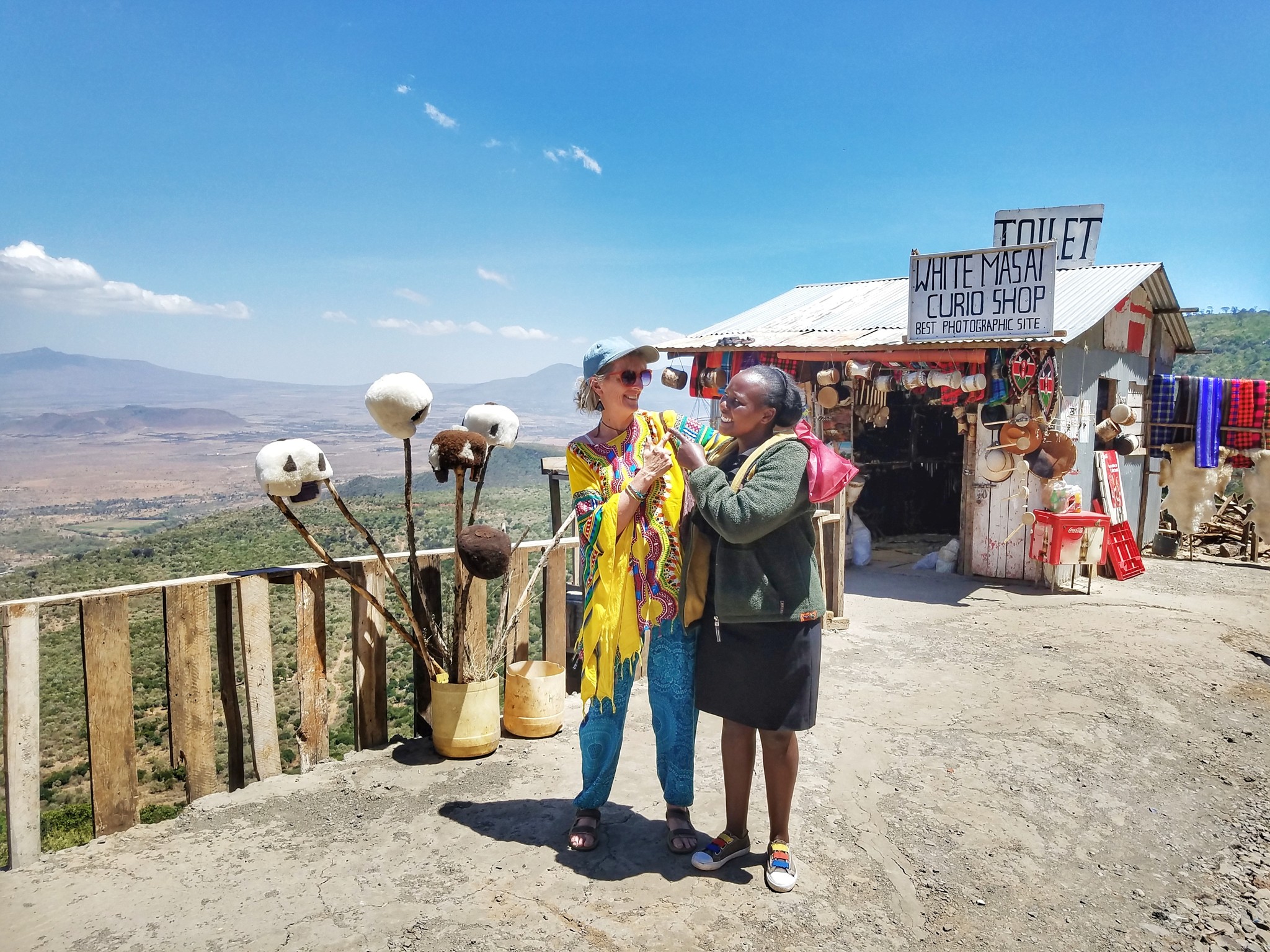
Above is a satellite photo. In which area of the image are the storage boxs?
[1029,509,1111,565]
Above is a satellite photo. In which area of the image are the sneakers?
[766,839,798,892]
[691,829,751,870]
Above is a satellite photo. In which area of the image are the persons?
[667,365,824,892]
[566,338,732,851]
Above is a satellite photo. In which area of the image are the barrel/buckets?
[1151,533,1180,557]
[502,660,566,739]
[429,671,500,757]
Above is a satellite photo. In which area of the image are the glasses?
[597,369,653,387]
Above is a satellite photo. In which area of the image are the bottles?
[1078,527,1089,562]
[1042,527,1048,554]
[1050,485,1082,513]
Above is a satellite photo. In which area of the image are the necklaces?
[601,417,624,432]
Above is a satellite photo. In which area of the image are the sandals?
[665,806,697,853]
[569,807,601,852]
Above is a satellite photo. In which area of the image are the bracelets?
[625,485,644,502]
[628,482,648,499]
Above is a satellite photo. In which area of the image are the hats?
[981,404,1007,430]
[583,339,659,381]
[1023,429,1077,480]
[977,441,1014,482]
[999,417,1042,455]
